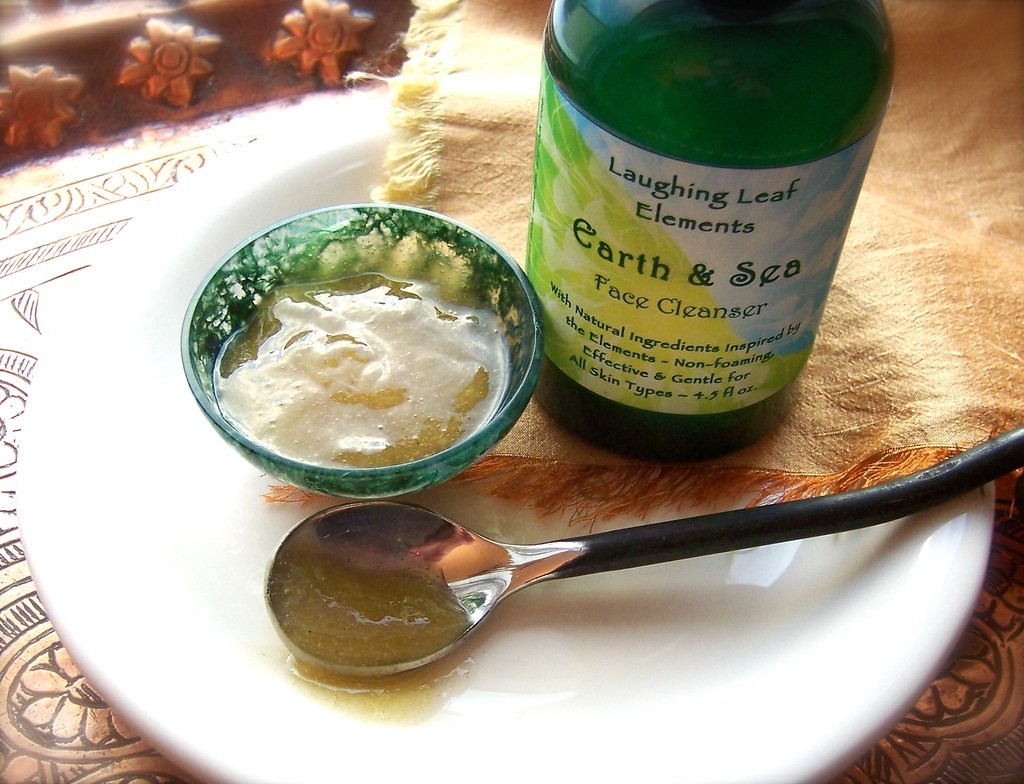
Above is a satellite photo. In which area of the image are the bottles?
[526,1,891,456]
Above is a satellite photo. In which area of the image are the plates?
[18,126,993,784]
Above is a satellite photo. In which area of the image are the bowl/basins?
[181,203,543,497]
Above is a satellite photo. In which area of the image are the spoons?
[265,426,1024,676]
